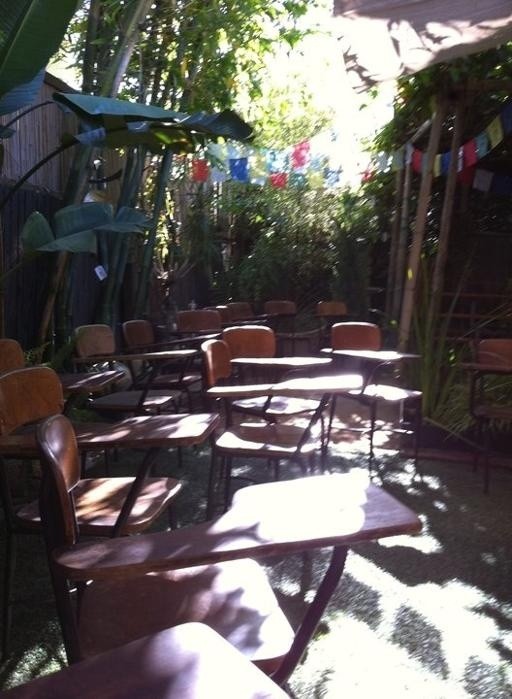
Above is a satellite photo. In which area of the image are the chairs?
[323,322,425,471]
[453,338,511,498]
[0,366,220,652]
[69,301,427,414]
[199,338,364,522]
[0,339,125,417]
[13,621,292,699]
[36,413,423,687]
[222,325,333,381]
